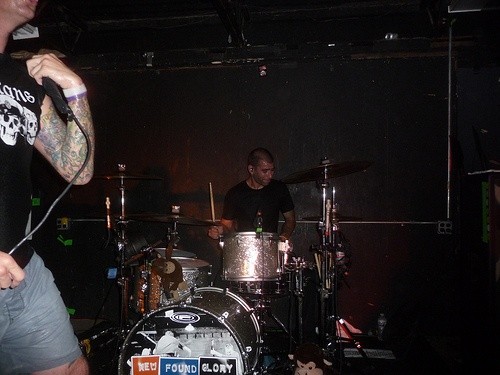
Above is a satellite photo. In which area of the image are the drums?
[121,247,197,310]
[119,286,262,375]
[220,231,290,282]
[131,256,212,316]
[227,250,295,297]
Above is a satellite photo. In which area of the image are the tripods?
[319,209,370,366]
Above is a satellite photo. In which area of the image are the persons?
[0,0,95,375]
[208,147,319,343]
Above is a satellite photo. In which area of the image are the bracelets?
[63,84,88,101]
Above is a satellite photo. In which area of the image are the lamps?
[210,51,225,64]
[142,51,155,67]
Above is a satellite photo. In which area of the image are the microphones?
[105,197,111,231]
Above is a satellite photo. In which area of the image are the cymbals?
[284,161,372,185]
[118,213,221,226]
[92,172,165,181]
[303,213,367,221]
